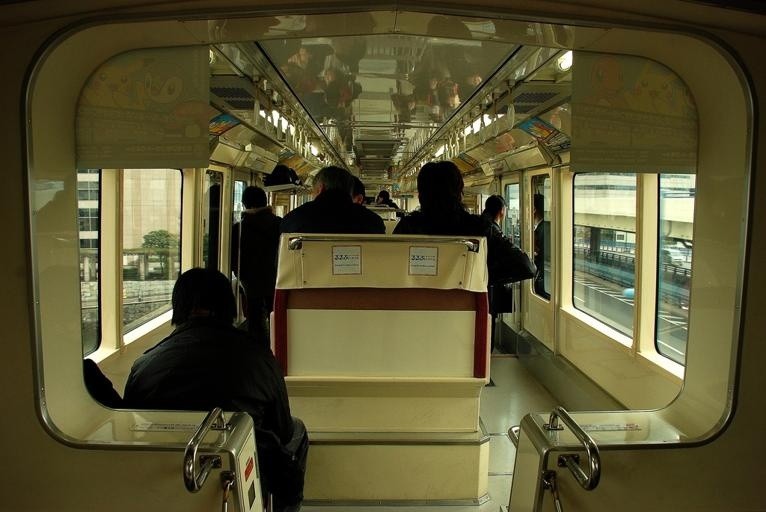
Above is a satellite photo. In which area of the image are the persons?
[531,193,551,300]
[121,265,309,511]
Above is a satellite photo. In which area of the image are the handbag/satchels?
[489,244,535,286]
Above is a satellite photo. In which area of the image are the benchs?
[270,229,492,436]
